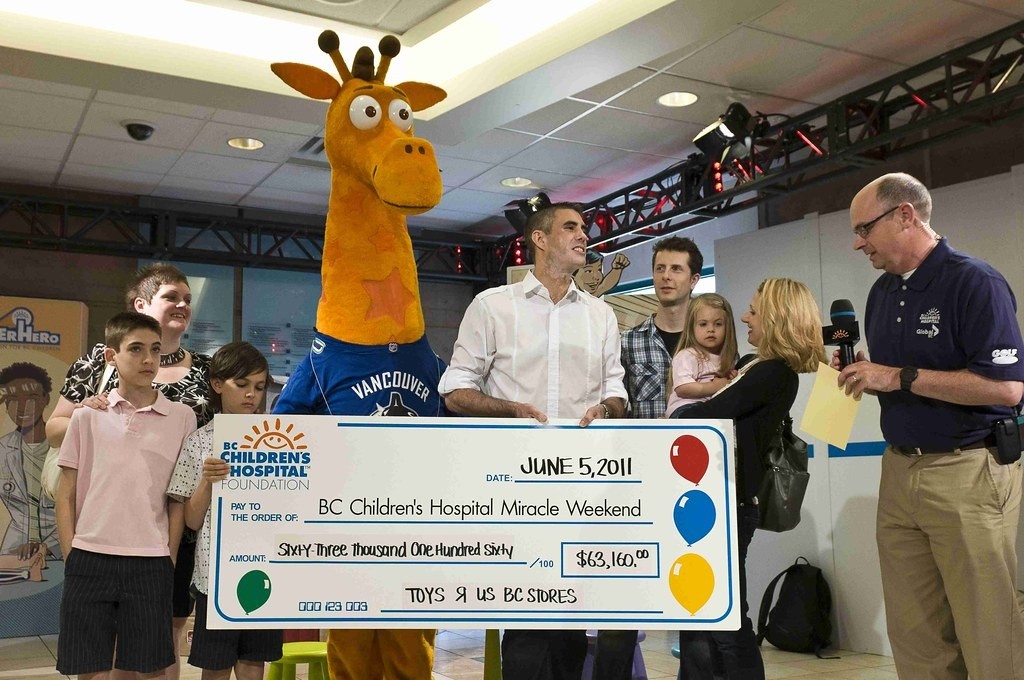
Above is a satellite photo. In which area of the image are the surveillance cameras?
[127,123,155,140]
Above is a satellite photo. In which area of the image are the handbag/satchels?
[757,418,809,532]
[40,363,116,503]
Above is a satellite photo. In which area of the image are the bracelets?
[602,404,609,419]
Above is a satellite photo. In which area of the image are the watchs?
[900,366,918,391]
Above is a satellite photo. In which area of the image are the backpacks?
[759,557,833,654]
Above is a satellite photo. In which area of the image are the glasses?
[854,205,899,240]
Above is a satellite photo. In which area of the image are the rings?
[853,376,856,381]
[94,393,98,396]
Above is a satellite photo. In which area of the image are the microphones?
[821,298,861,370]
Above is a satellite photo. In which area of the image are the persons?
[45,263,219,680]
[56,312,198,680]
[665,294,738,420]
[829,172,1024,680]
[437,201,629,679]
[165,341,283,680]
[659,278,826,680]
[590,236,713,679]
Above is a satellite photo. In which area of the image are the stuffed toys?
[269,30,449,680]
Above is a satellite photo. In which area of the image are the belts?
[893,433,996,457]
